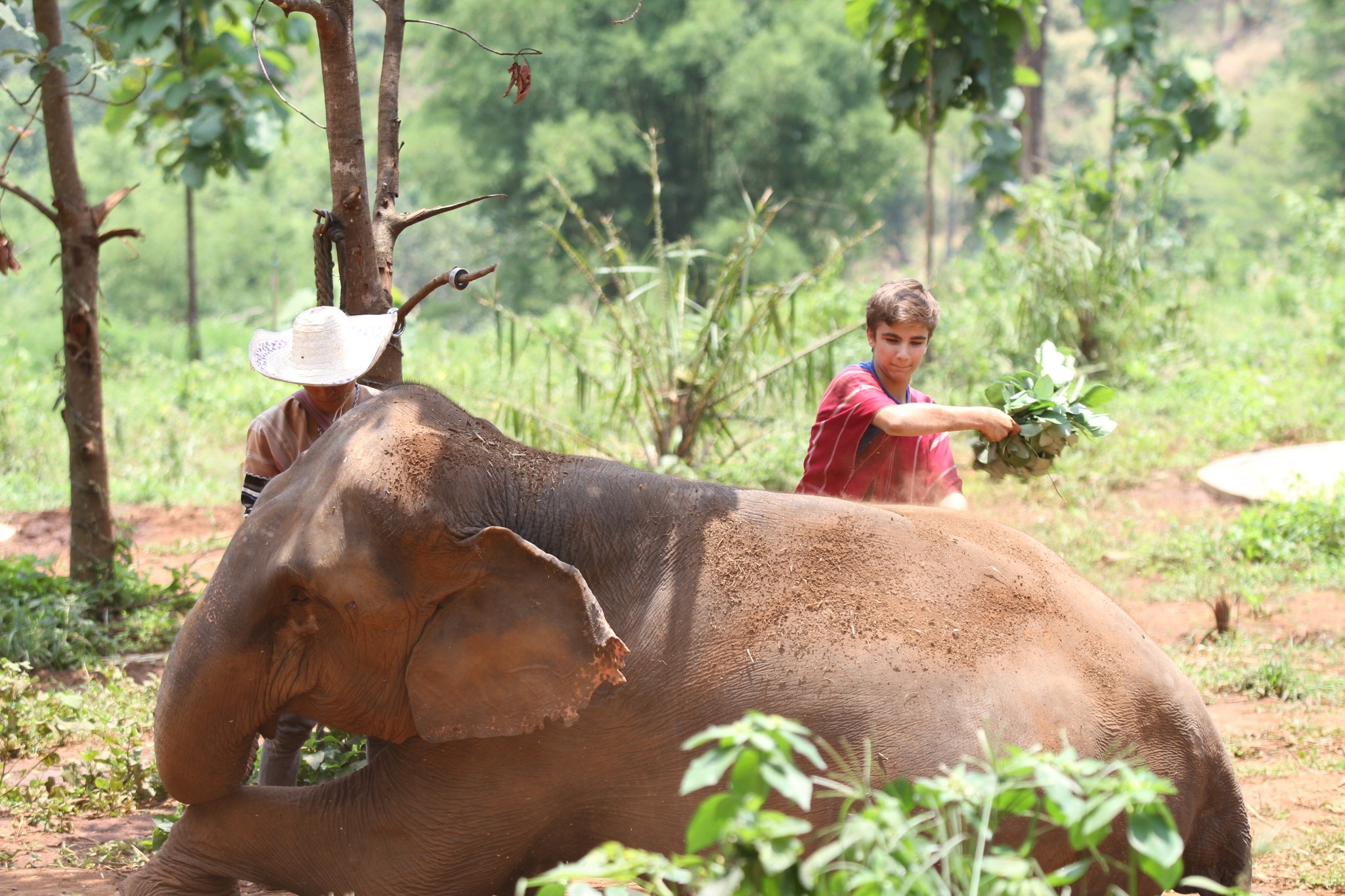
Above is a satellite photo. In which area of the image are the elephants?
[120,382,1251,896]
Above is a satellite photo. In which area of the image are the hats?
[249,306,398,385]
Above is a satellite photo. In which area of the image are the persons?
[796,277,1022,509]
[240,306,398,785]
